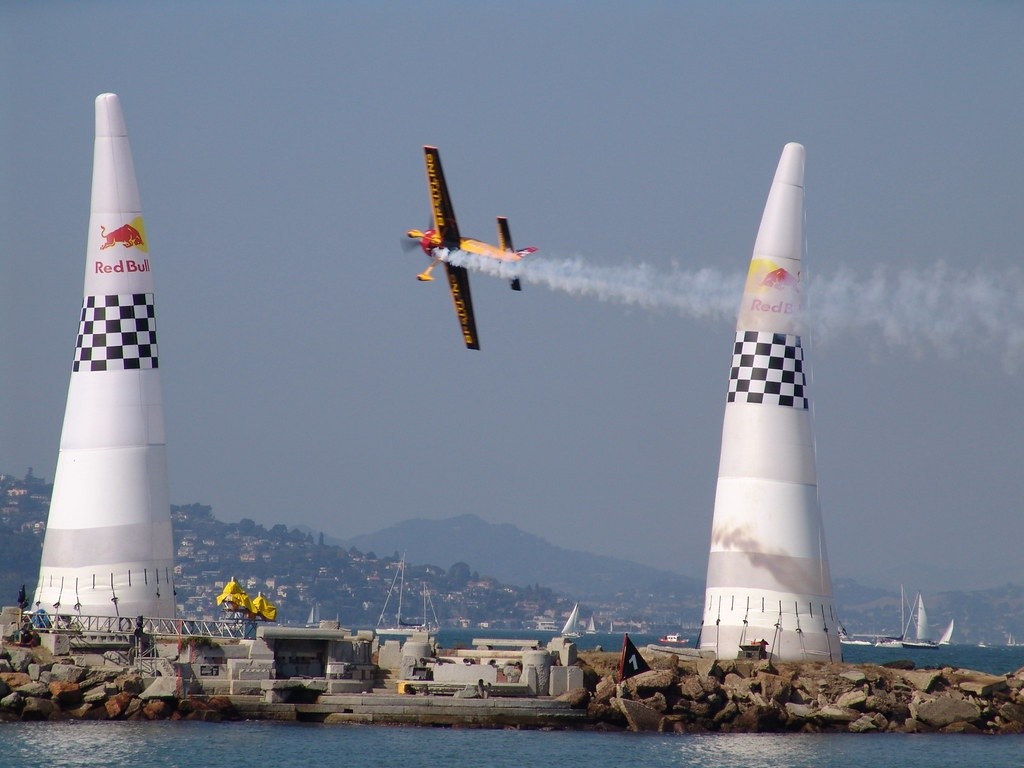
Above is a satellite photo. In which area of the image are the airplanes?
[407,144,539,351]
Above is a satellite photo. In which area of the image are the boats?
[657,631,690,644]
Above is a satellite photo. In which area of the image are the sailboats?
[938,618,954,645]
[839,584,904,648]
[560,600,585,639]
[585,615,600,634]
[977,632,1024,648]
[375,550,441,635]
[895,589,940,649]
[305,602,321,628]
[607,621,614,635]
